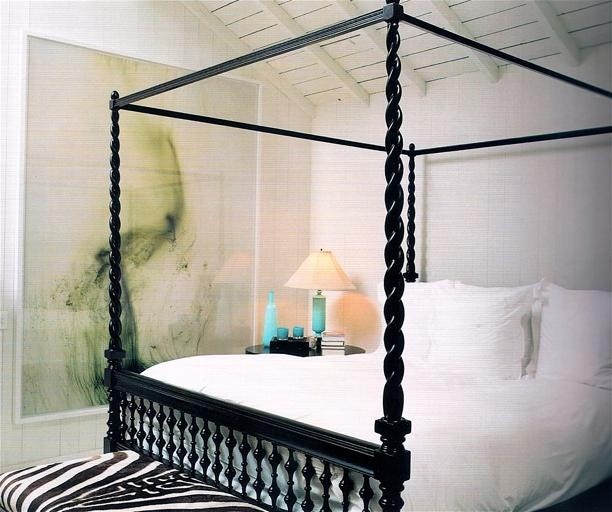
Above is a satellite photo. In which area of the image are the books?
[320,330,346,356]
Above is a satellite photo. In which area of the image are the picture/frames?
[12,32,263,427]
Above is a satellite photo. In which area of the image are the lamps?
[283,246,357,349]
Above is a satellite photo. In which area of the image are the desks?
[245,344,367,360]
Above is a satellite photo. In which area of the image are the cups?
[292,327,304,339]
[276,328,289,341]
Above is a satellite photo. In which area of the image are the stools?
[1,447,270,512]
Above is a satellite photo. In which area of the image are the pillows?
[375,279,611,389]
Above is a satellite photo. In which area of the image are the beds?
[102,0,612,512]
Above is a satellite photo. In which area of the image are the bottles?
[261,290,279,348]
